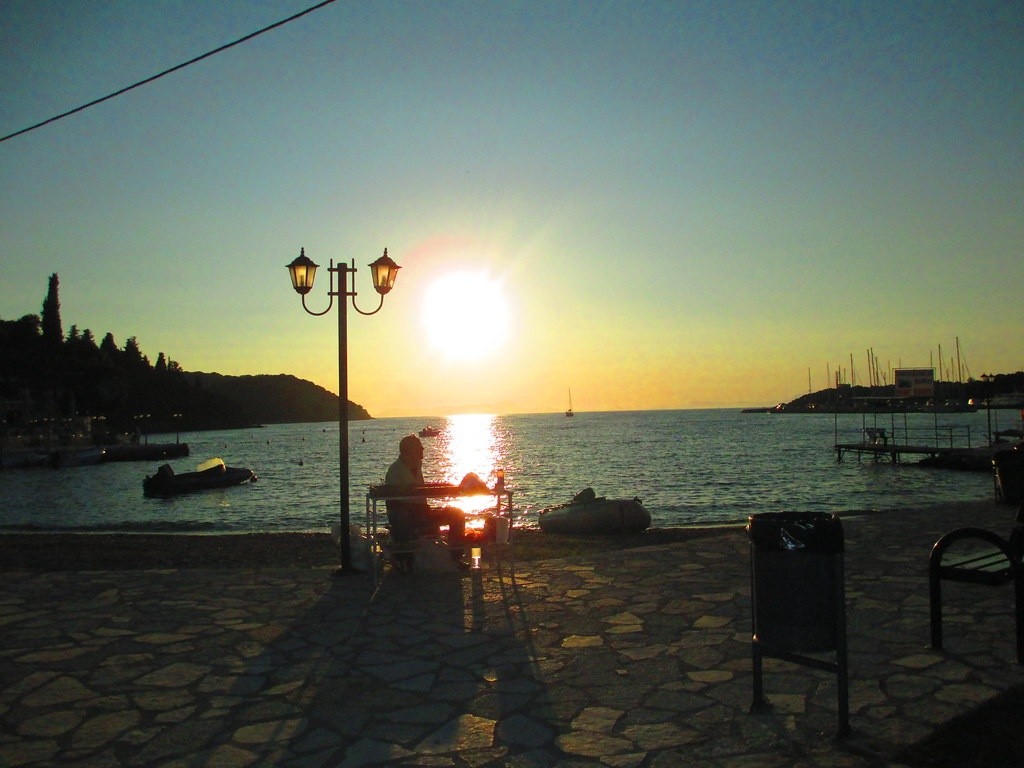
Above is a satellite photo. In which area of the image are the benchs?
[925,494,1024,667]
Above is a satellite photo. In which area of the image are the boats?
[538,487,651,535]
[419,425,442,437]
[565,409,573,416]
[143,464,253,498]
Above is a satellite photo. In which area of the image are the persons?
[385,436,471,570]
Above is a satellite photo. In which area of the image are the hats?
[399,436,427,453]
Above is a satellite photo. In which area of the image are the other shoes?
[457,553,468,564]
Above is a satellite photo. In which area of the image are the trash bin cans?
[748,510,845,653]
[992,450,1024,504]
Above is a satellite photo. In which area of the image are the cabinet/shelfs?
[365,487,514,579]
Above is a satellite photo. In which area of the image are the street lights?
[139,413,183,445]
[285,247,403,578]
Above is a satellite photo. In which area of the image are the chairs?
[865,427,892,447]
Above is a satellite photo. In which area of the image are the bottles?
[498,469,504,488]
